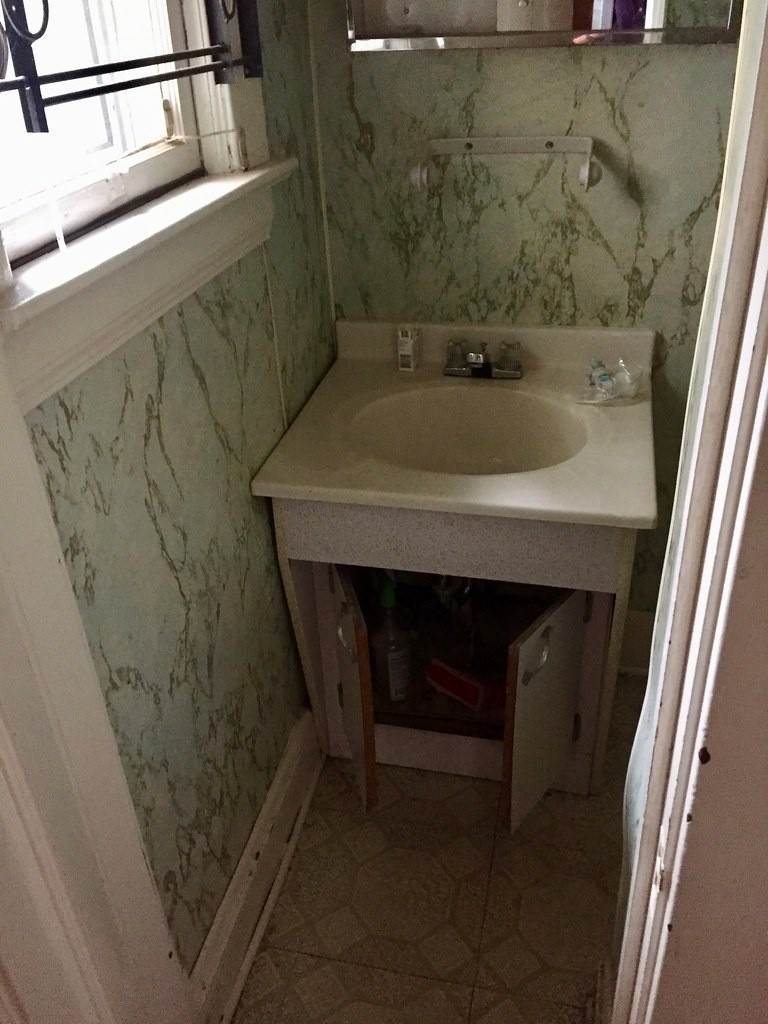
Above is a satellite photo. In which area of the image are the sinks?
[334,377,612,475]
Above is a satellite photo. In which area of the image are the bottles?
[371,586,419,706]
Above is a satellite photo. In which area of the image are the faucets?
[465,342,493,379]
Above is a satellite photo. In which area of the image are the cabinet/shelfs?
[295,563,608,838]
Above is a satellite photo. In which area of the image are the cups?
[666,0,734,48]
[610,0,643,45]
